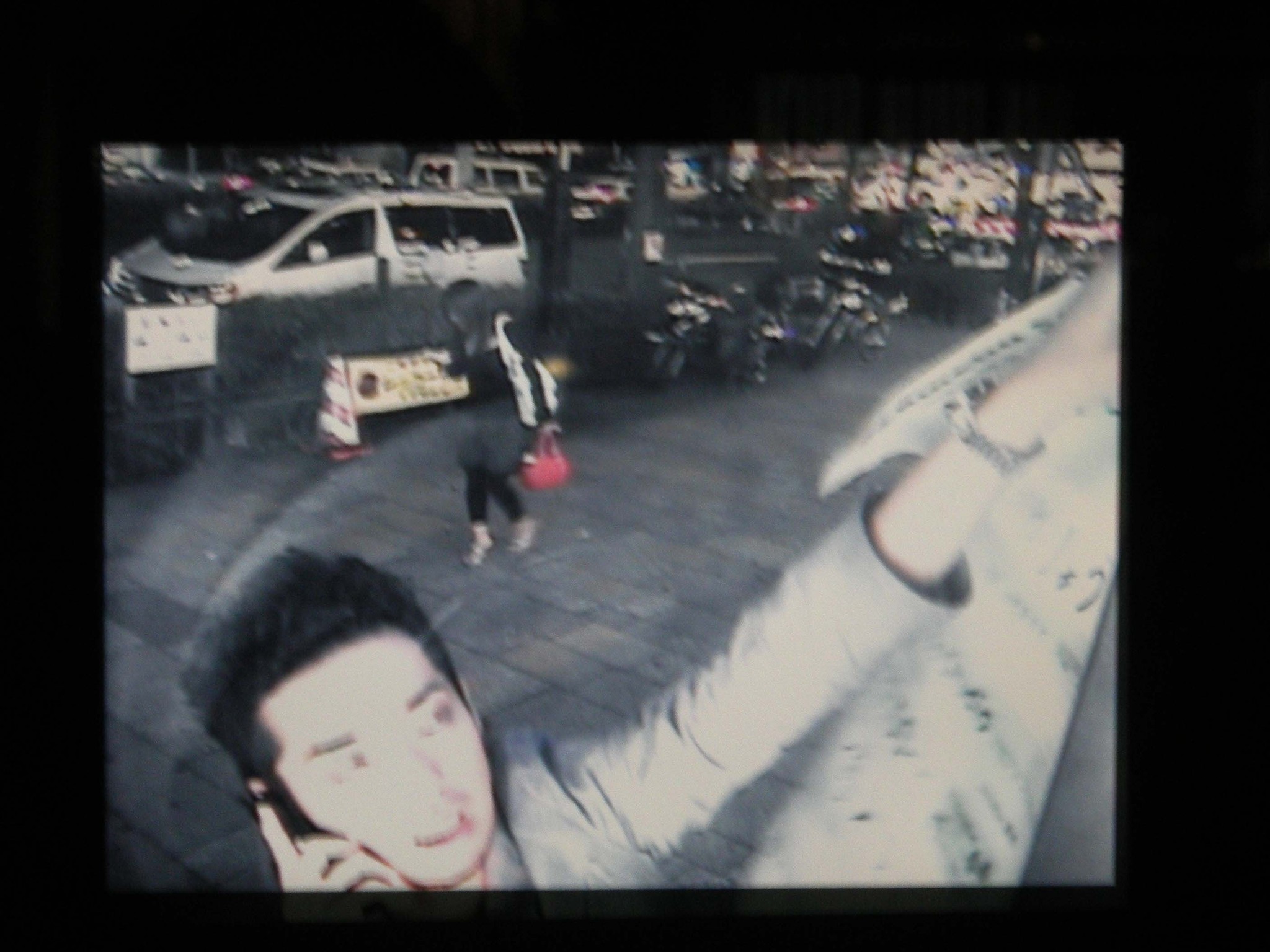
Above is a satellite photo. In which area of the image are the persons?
[170,244,1120,919]
[413,278,564,570]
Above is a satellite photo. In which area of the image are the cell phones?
[229,785,299,858]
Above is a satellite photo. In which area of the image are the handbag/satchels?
[493,313,560,427]
[514,429,572,490]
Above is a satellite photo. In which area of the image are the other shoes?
[508,516,536,556]
[463,533,495,566]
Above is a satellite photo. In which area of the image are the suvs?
[104,189,532,403]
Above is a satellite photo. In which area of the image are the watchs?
[939,378,1045,476]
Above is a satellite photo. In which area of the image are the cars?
[103,144,715,234]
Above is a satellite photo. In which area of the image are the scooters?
[637,224,911,392]
